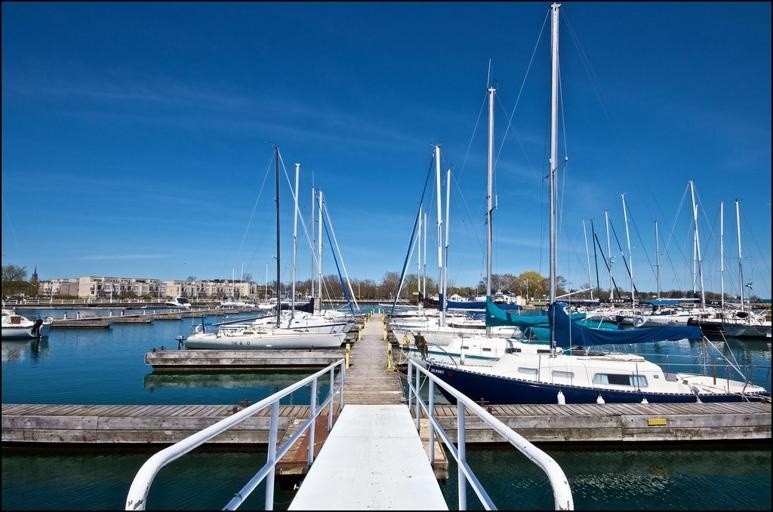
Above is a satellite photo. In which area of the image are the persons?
[415,333,428,361]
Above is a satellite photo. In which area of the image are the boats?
[1,306,52,341]
[42,311,108,328]
[106,313,150,324]
